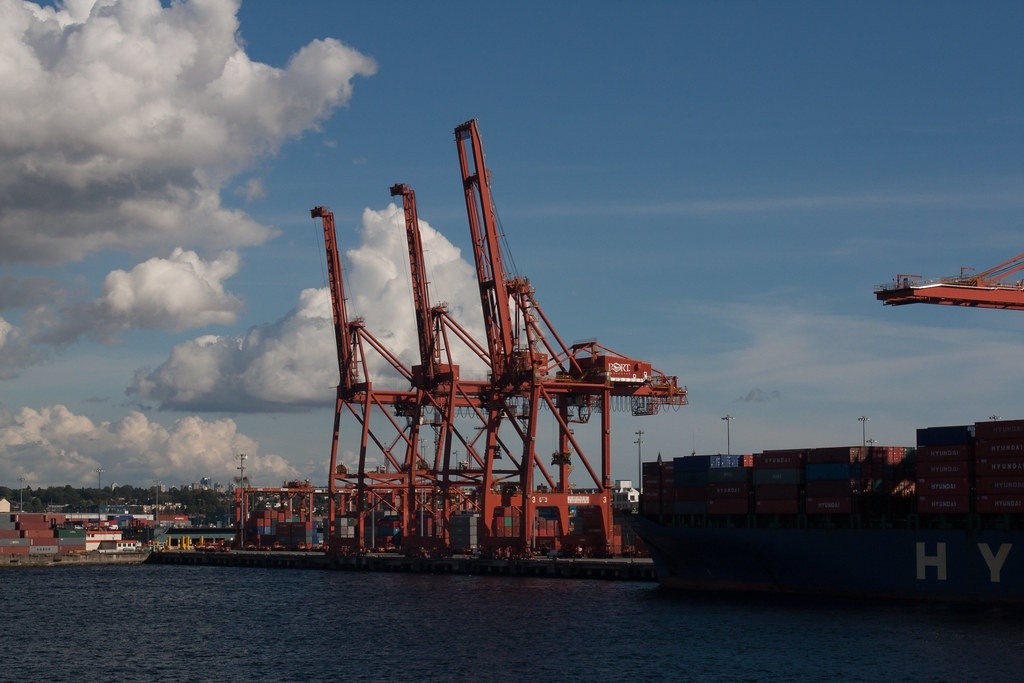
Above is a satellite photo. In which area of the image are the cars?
[73,524,84,530]
[88,526,99,531]
[195,541,231,553]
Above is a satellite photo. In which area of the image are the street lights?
[19,477,25,513]
[96,466,106,528]
[634,430,645,495]
[238,453,248,550]
[721,415,735,455]
[857,416,870,446]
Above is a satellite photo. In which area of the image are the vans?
[109,525,119,531]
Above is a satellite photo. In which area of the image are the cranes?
[310,183,556,555]
[455,117,687,553]
[871,251,1023,311]
